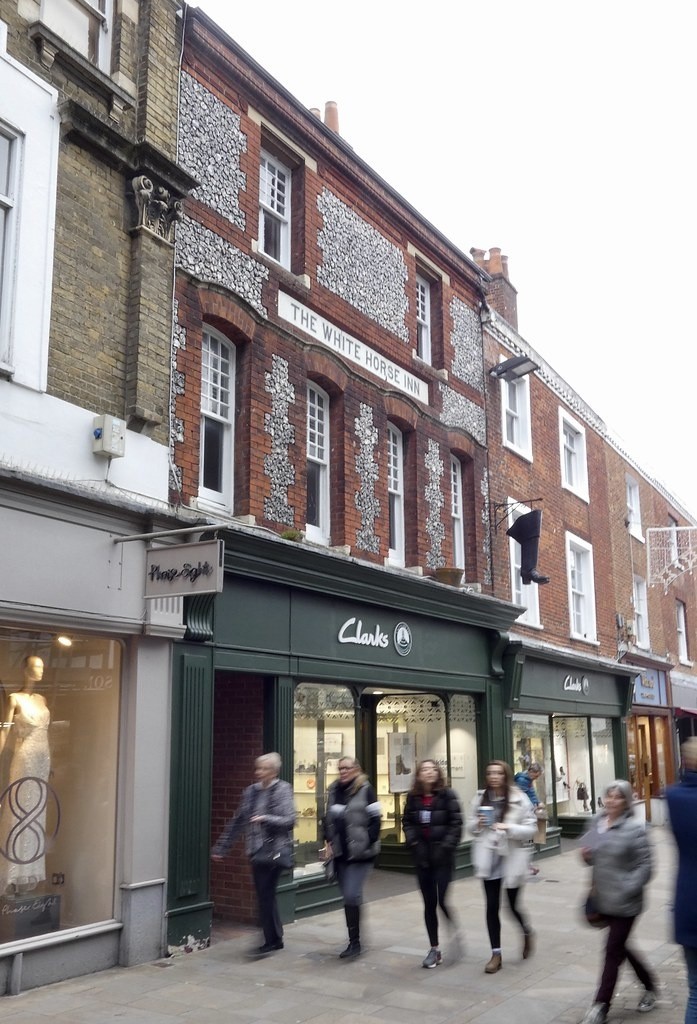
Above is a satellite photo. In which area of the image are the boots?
[337,904,359,958]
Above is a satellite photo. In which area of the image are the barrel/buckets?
[437,568,464,587]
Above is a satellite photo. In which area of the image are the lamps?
[489,356,539,383]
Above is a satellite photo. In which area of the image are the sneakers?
[636,991,662,1011]
[421,948,443,968]
[581,998,606,1022]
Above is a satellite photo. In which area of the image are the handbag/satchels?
[533,804,551,847]
[583,890,611,927]
[322,855,338,882]
[471,827,509,879]
[247,817,295,871]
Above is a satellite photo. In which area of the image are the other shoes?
[522,926,535,959]
[487,951,503,973]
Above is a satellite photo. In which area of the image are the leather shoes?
[261,940,283,951]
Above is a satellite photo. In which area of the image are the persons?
[466,760,540,973]
[666,737,697,1024]
[402,759,463,968]
[579,780,660,1024]
[0,656,51,897]
[210,752,297,953]
[322,756,381,958]
[515,764,541,806]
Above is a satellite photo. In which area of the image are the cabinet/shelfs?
[293,771,406,847]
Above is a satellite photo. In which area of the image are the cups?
[477,806,494,825]
